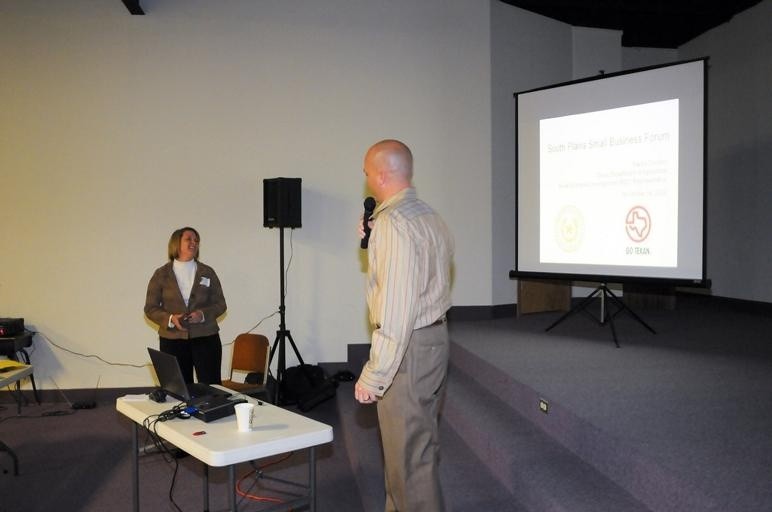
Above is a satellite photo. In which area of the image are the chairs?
[221,334,273,405]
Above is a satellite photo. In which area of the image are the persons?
[354,141,450,511]
[143,227,227,459]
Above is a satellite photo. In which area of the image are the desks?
[0,333,40,415]
[0,359,33,476]
[116,383,334,512]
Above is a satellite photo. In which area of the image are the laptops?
[146,346,232,403]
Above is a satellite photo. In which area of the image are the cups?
[234,403,256,432]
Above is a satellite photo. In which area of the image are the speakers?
[262,177,303,231]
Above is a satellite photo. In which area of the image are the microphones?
[358,195,376,252]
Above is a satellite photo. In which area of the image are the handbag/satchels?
[296,369,354,412]
[284,364,324,400]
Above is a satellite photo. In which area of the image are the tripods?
[269,240,305,407]
[543,287,658,349]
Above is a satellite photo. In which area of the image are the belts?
[431,317,446,327]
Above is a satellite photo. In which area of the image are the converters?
[190,399,236,422]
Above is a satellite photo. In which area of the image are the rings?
[364,397,370,401]
